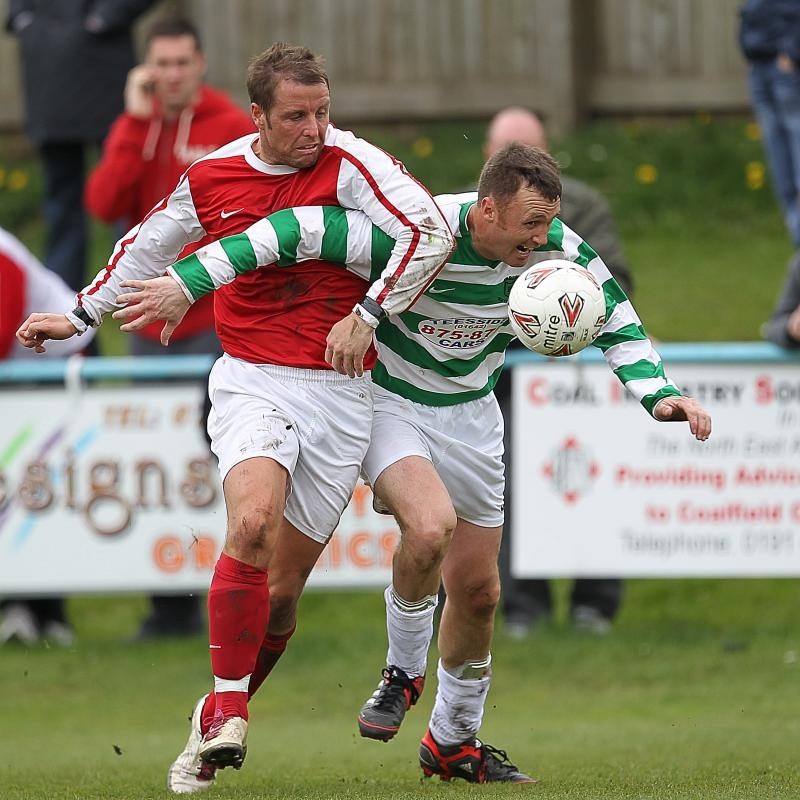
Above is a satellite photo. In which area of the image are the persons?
[110,140,713,783]
[0,224,96,648]
[483,105,637,637]
[735,1,800,249]
[14,42,457,794]
[83,15,259,640]
[762,252,800,350]
[0,0,160,357]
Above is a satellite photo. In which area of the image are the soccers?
[507,259,607,357]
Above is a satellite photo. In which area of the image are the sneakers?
[419,728,537,785]
[167,695,217,794]
[358,665,426,743]
[199,714,248,769]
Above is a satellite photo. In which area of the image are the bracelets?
[352,295,387,330]
[73,306,95,328]
